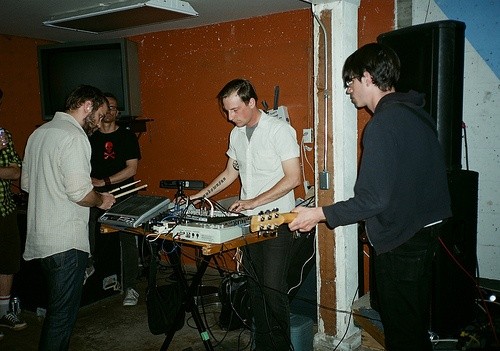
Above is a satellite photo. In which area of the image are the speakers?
[374,18,466,168]
[431,166,479,330]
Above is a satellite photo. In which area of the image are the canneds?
[0,128,9,146]
[11,297,20,315]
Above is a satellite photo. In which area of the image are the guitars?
[250,207,370,242]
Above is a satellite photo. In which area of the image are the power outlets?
[303,128,314,144]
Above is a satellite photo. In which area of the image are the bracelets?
[103,177,111,186]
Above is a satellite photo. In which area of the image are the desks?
[99,223,292,351]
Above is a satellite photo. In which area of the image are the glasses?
[346,75,367,87]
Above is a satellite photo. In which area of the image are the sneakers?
[0,310,27,331]
[122,288,140,306]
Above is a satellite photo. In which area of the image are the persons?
[0,126,28,330]
[20,85,117,351]
[288,42,454,351]
[88,93,139,304]
[172,79,303,351]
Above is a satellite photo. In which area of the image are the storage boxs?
[290,313,314,351]
